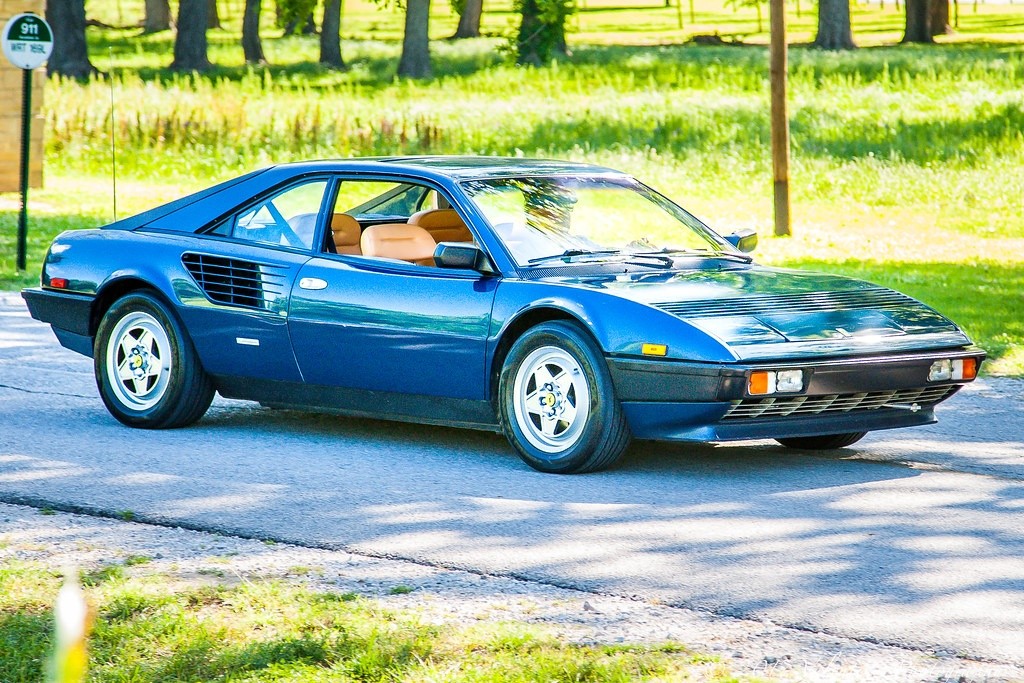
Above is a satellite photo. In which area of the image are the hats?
[524,183,578,205]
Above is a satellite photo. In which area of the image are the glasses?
[558,205,574,213]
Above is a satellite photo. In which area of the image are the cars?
[22,157,988,475]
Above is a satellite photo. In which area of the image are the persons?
[519,186,647,257]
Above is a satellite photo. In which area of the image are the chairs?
[279,209,474,267]
